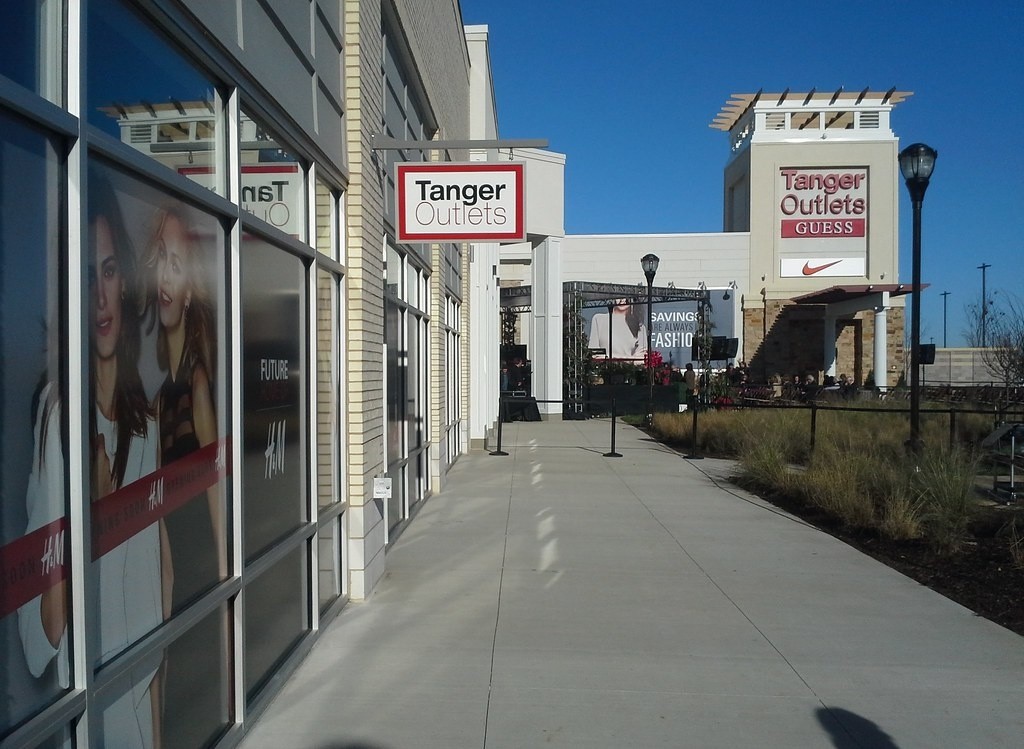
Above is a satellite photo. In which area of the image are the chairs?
[738,388,1024,406]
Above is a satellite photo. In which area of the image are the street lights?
[603,301,624,457]
[722,281,746,362]
[760,275,767,337]
[897,143,937,455]
[640,254,660,415]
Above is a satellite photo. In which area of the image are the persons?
[794,375,801,385]
[16,167,223,749]
[587,297,648,365]
[806,375,814,384]
[836,374,852,387]
[684,363,695,404]
[510,357,525,420]
[500,364,513,423]
[823,375,836,388]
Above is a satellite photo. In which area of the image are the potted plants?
[606,361,636,384]
